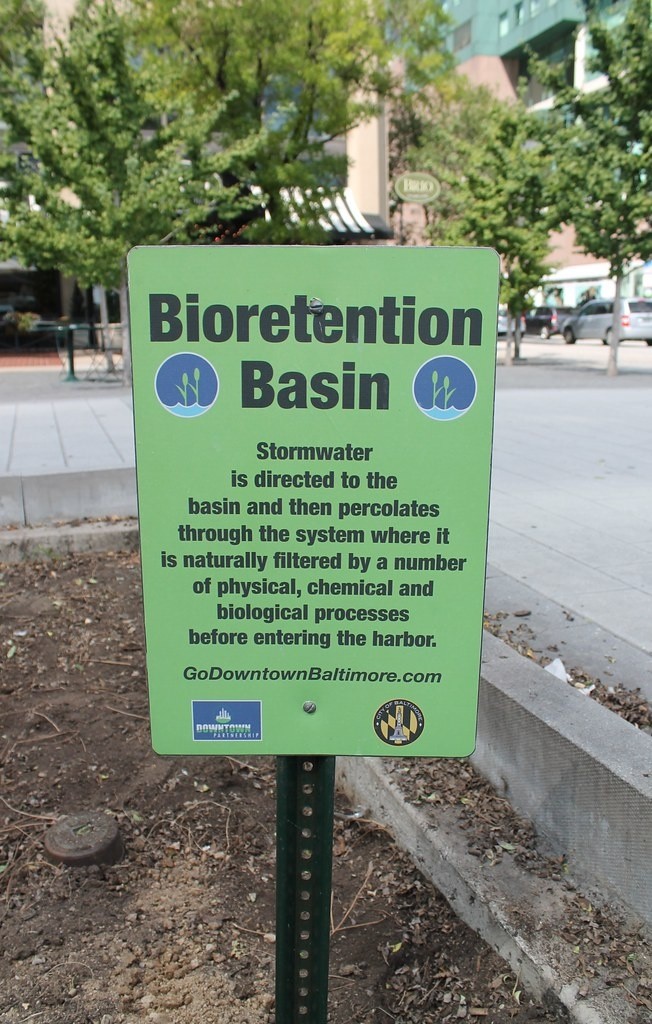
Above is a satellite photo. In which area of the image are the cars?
[497,302,527,339]
[522,304,578,340]
[560,295,652,347]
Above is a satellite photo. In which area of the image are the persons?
[13,284,37,313]
